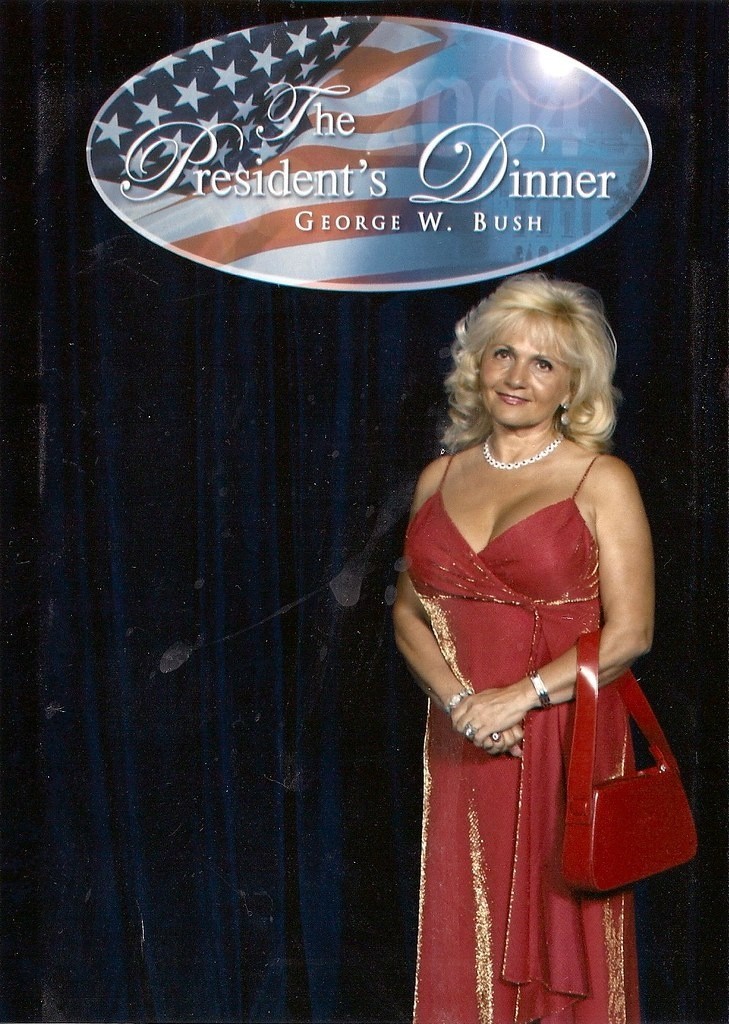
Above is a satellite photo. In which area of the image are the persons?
[394,273,652,1024]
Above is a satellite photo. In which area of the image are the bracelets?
[443,688,473,715]
[525,668,552,708]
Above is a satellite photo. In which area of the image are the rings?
[491,731,501,742]
[462,723,479,739]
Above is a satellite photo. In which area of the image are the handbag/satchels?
[561,631,698,892]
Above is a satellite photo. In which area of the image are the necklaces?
[483,433,566,471]
